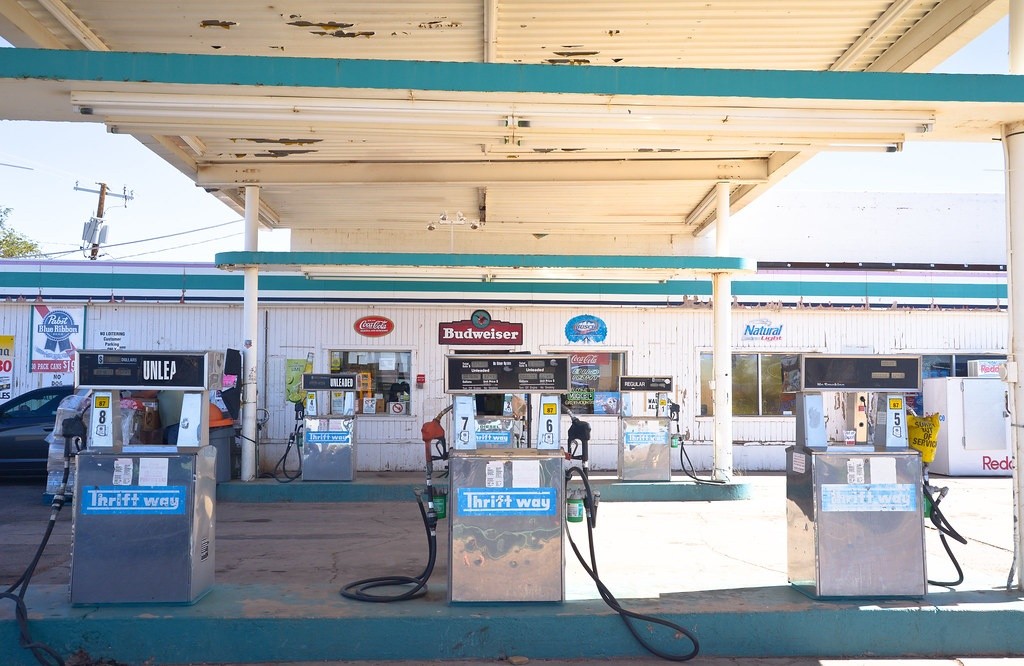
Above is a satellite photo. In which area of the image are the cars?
[0,385,74,480]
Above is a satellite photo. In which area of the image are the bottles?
[298,352,314,408]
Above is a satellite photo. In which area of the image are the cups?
[844,430,856,445]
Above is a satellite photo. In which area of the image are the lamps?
[70,89,938,153]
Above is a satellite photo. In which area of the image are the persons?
[389,382,410,402]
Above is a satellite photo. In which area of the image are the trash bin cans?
[209,402,236,483]
